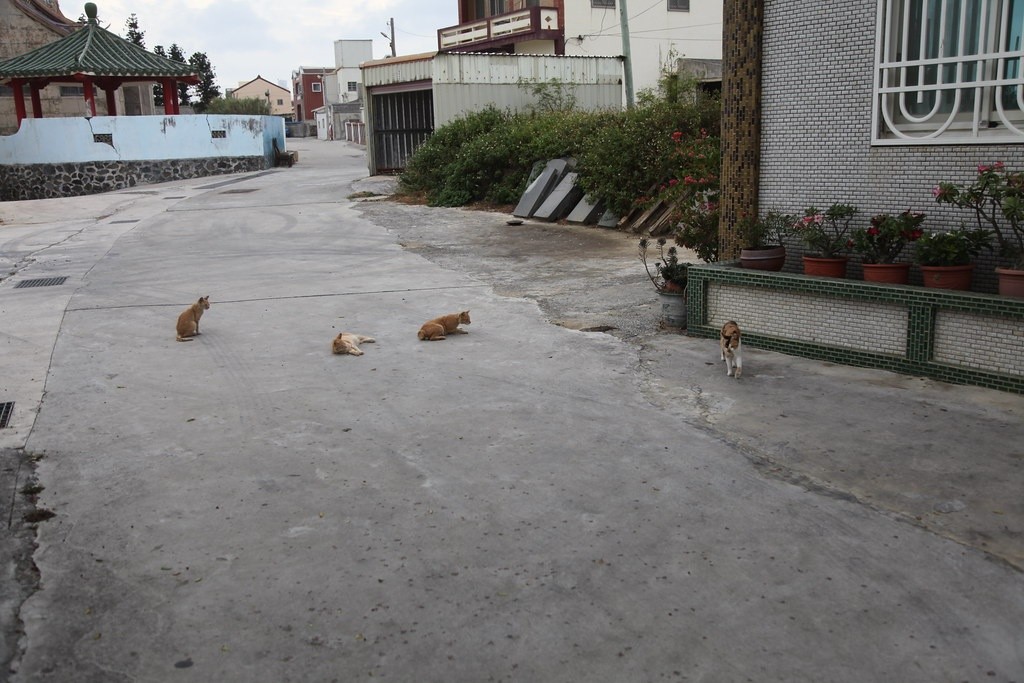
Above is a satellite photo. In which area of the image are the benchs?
[271,138,294,168]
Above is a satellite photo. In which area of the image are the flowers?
[636,128,1024,259]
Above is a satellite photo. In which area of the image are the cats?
[332,333,376,356]
[720,321,743,380]
[417,310,471,341]
[176,296,210,342]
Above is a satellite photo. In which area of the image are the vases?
[861,262,912,284]
[995,267,1023,296]
[921,262,978,288]
[741,246,786,272]
[801,253,847,277]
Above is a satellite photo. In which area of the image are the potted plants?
[639,239,696,328]
